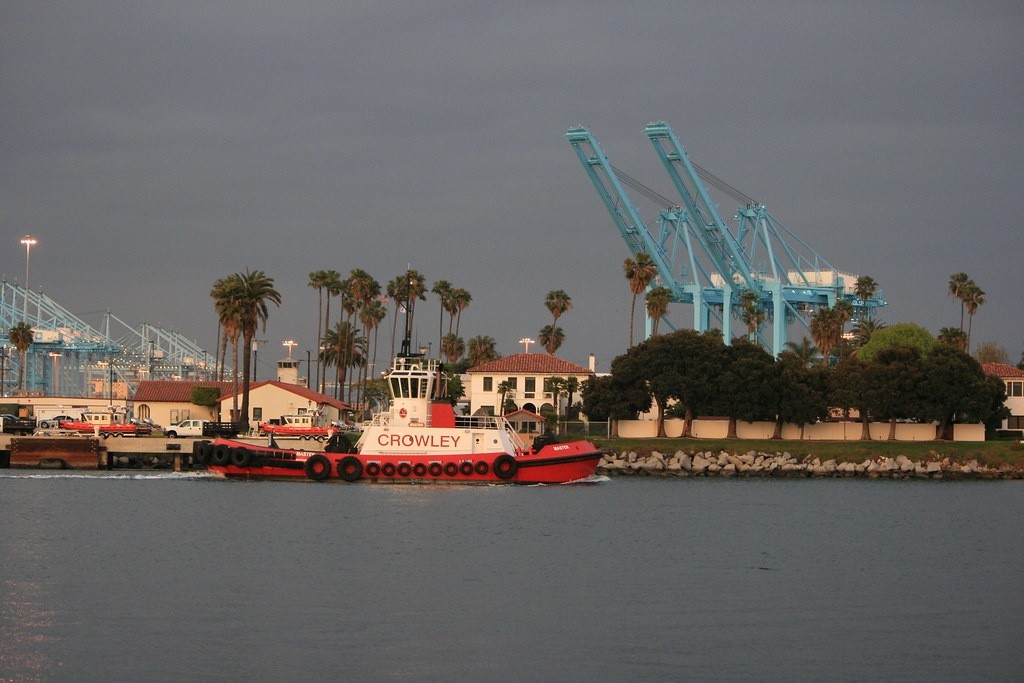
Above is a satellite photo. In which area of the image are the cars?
[163,420,210,438]
[38,415,79,429]
[0,413,36,436]
[326,419,357,432]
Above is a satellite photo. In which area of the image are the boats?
[260,414,339,444]
[193,358,604,485]
[59,411,136,439]
